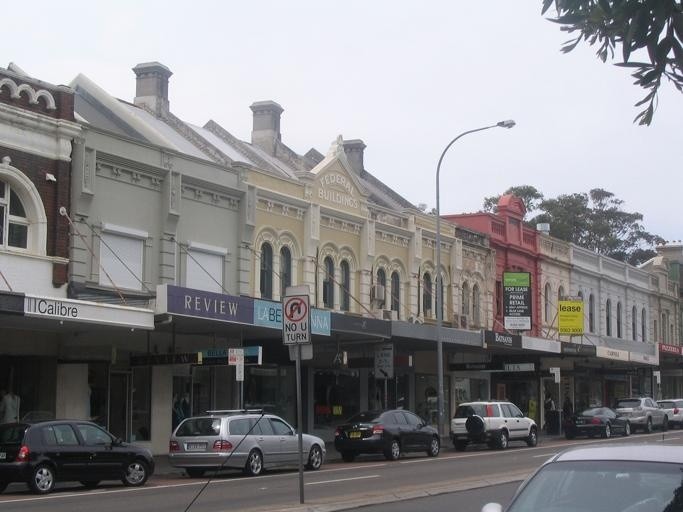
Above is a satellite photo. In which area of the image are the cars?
[480,442,682,512]
[334,410,440,462]
[566,405,630,441]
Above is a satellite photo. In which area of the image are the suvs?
[613,396,667,434]
[655,398,682,429]
[449,399,539,452]
[0,419,156,492]
[170,409,325,476]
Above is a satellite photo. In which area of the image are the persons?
[562,396,573,420]
[0,390,21,424]
[173,392,191,436]
[543,392,555,435]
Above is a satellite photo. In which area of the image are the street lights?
[434,117,514,437]
